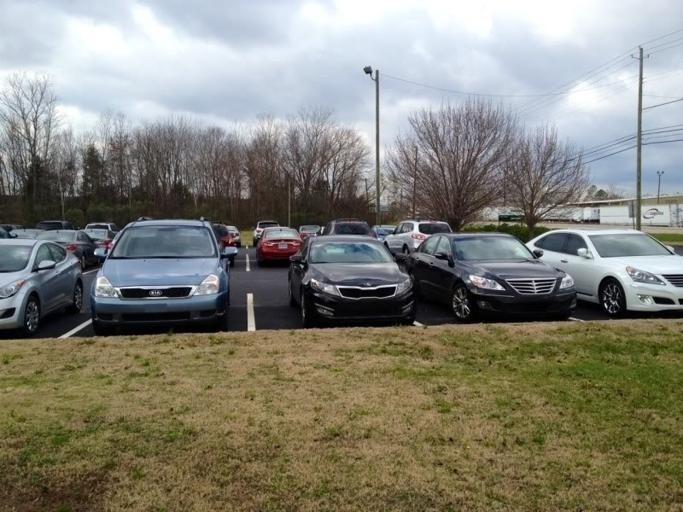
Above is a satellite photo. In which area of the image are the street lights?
[364,66,382,228]
[657,168,665,204]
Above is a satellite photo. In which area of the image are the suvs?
[382,220,454,259]
[89,216,237,333]
[318,218,377,241]
[253,220,280,246]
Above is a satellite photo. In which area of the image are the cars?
[256,227,304,265]
[0,239,84,335]
[299,225,321,239]
[526,226,682,318]
[370,224,397,241]
[288,234,417,328]
[404,231,578,323]
[1,214,242,270]
